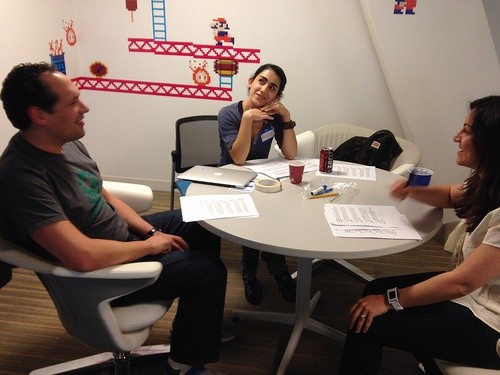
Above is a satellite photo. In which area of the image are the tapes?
[254,178,280,192]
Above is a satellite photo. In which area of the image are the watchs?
[145,225,161,239]
[388,287,404,311]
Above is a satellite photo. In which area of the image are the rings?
[360,314,366,320]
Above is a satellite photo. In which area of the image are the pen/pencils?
[249,107,275,130]
[405,169,416,186]
[308,193,338,199]
[315,188,333,195]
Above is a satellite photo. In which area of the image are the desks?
[186,157,444,375]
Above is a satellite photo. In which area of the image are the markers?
[311,185,327,195]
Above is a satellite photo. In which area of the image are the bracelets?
[282,120,296,130]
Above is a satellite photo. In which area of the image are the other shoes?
[161,362,226,375]
[245,278,263,304]
[220,325,241,345]
[274,272,297,303]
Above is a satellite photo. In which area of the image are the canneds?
[319,147,334,173]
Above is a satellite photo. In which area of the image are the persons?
[340,95,500,375]
[1,63,237,375]
[219,64,296,303]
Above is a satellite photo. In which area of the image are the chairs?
[169,115,222,210]
[273,126,422,284]
[0,180,174,375]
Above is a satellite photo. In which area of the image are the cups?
[289,161,305,184]
[407,166,434,186]
